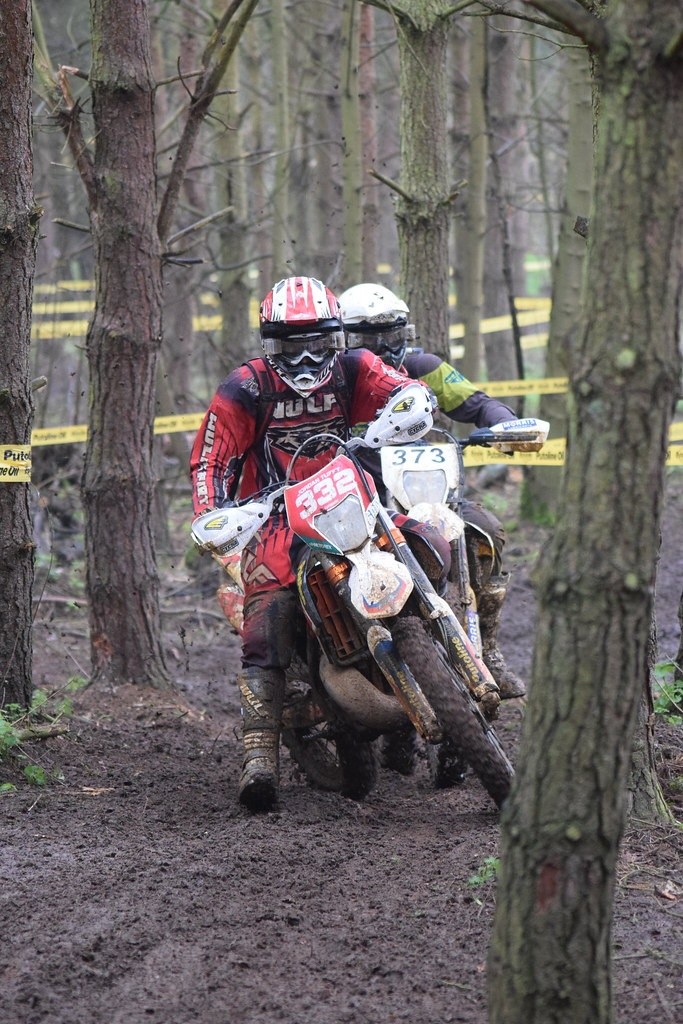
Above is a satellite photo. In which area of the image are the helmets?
[259,276,343,399]
[339,283,412,371]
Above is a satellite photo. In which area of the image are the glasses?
[259,330,346,365]
[348,324,410,357]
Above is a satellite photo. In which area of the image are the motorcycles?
[190,382,524,810]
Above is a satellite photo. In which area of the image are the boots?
[479,573,526,699]
[237,666,285,813]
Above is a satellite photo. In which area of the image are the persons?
[341,282,550,698]
[191,277,452,807]
